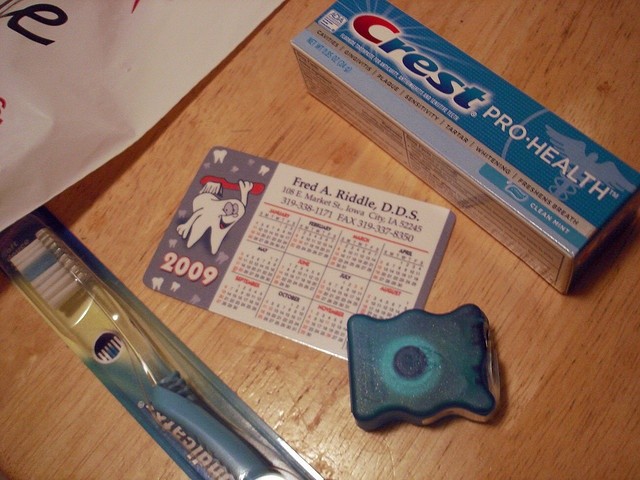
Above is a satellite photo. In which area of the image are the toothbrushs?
[10,224,287,479]
